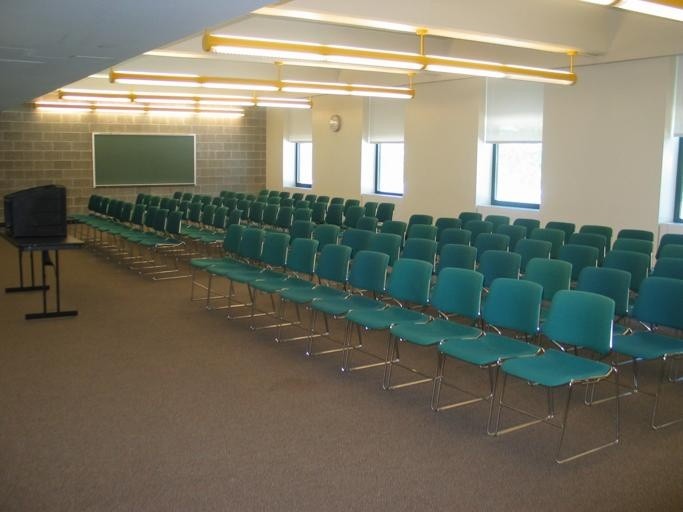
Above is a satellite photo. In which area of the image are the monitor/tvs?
[3,184,68,240]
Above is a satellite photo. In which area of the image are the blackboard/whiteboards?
[92,133,196,188]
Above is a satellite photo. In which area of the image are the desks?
[0,221,86,322]
